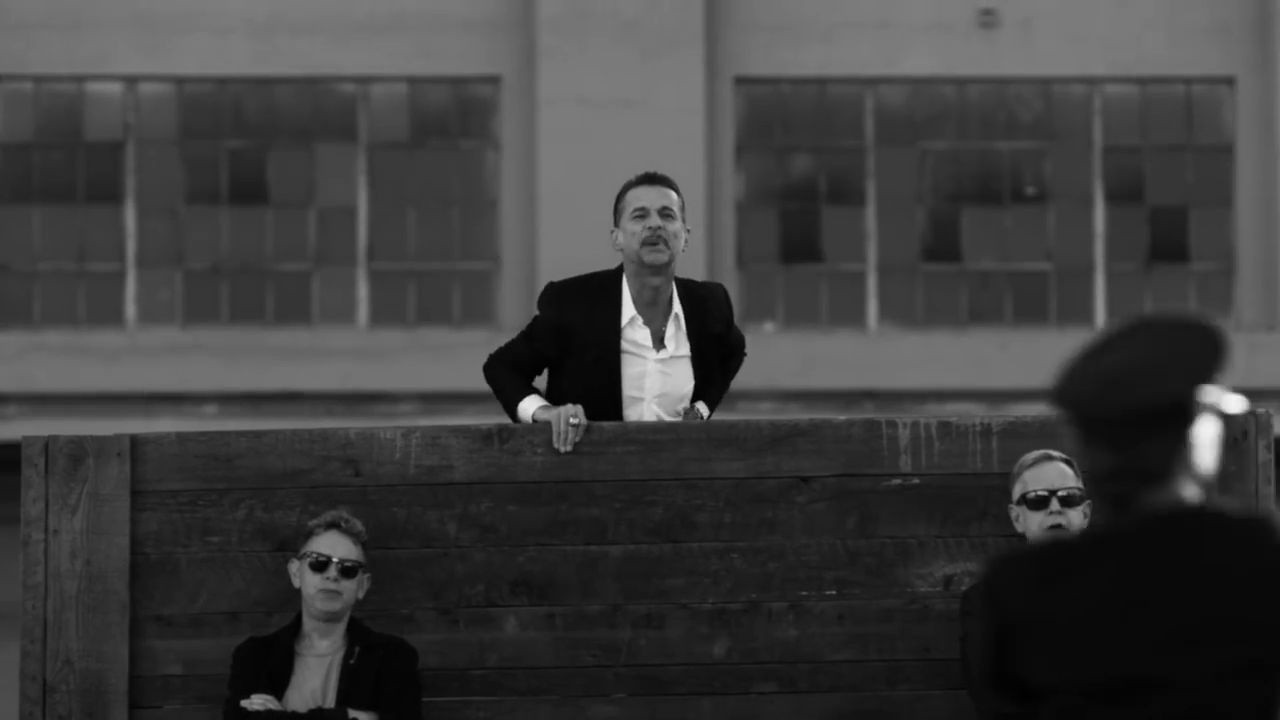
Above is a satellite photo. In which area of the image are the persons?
[224,512,419,720]
[1007,449,1095,540]
[483,170,747,455]
[958,316,1280,720]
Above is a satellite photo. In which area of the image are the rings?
[567,416,580,427]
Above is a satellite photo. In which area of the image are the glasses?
[297,551,375,580]
[1016,487,1090,511]
